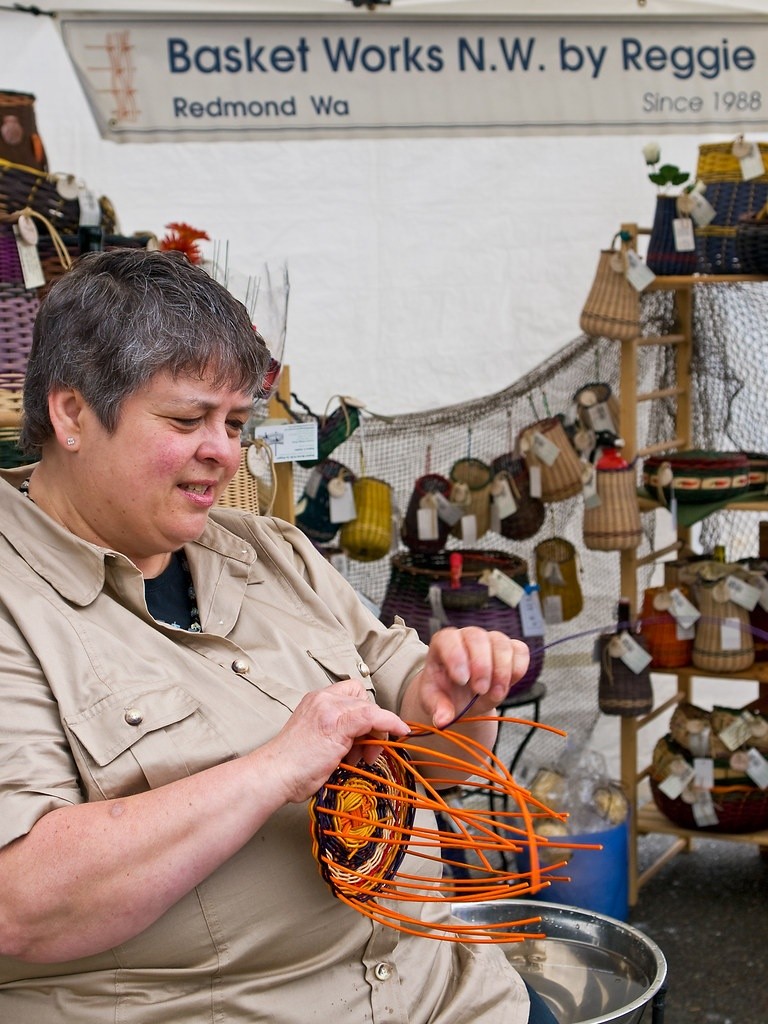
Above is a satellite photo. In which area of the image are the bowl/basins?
[450,899,668,1024]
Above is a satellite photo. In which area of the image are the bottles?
[714,544,725,564]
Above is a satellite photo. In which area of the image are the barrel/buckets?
[511,793,631,925]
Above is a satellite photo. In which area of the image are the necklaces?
[19,477,167,577]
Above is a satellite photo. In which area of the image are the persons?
[0,248,560,1024]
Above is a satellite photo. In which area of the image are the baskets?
[0,92,767,831]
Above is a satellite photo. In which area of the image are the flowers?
[639,136,696,197]
[156,221,212,268]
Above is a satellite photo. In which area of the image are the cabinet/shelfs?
[619,221,768,909]
